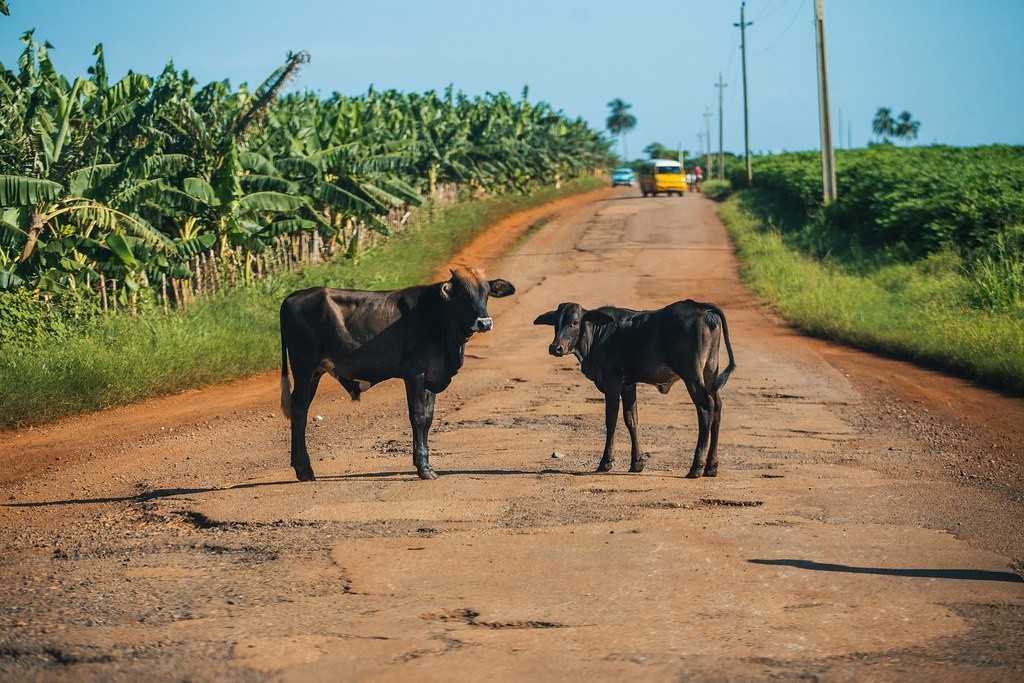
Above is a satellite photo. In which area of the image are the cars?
[610,168,637,187]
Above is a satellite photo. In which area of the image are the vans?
[638,158,687,197]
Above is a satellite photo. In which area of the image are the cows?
[532,297,737,480]
[278,266,516,483]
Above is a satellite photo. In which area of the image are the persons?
[685,166,704,194]
